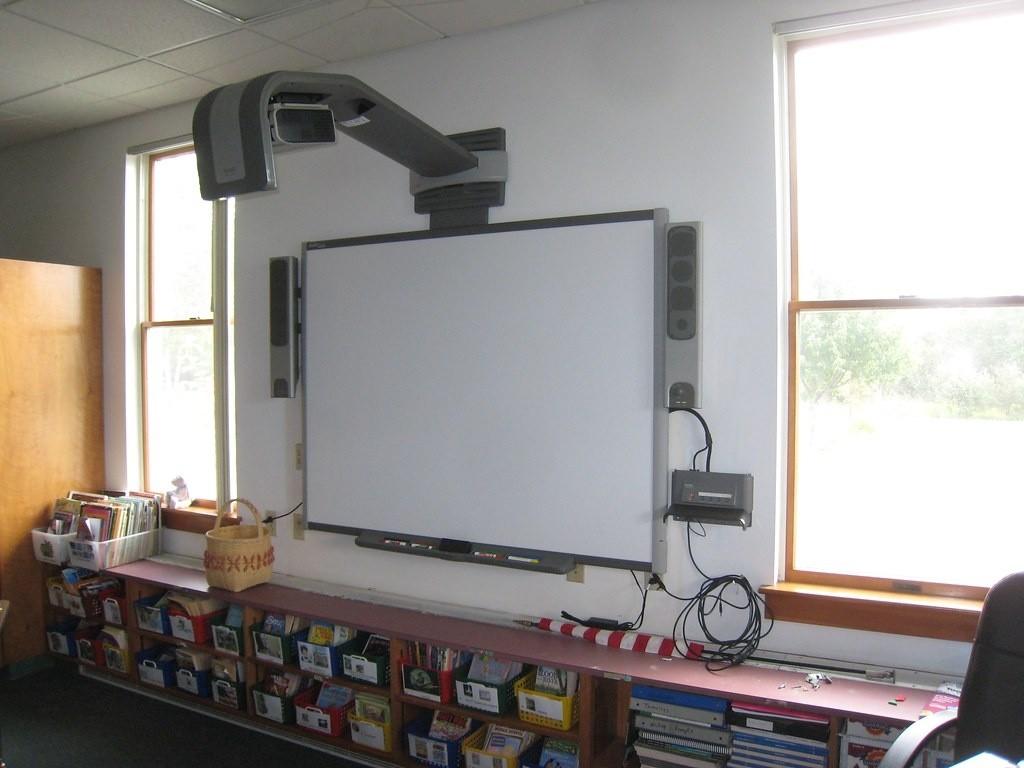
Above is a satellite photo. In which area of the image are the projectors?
[268,108,337,146]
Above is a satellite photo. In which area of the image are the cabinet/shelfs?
[38,550,935,767]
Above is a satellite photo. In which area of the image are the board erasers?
[437,537,471,554]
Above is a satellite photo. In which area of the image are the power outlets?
[265,511,277,537]
[295,513,305,541]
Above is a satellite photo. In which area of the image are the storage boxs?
[406,711,482,767]
[347,706,393,753]
[69,528,164,570]
[250,679,306,724]
[339,637,390,687]
[398,653,457,704]
[135,643,177,688]
[518,737,579,767]
[514,666,581,731]
[294,626,368,680]
[451,664,532,717]
[211,615,244,657]
[46,617,80,658]
[29,528,76,567]
[460,722,543,767]
[98,588,128,629]
[74,629,105,668]
[166,608,228,644]
[46,575,69,608]
[66,592,104,619]
[174,664,212,698]
[101,641,130,674]
[249,621,309,667]
[292,683,354,737]
[210,675,246,710]
[133,593,170,635]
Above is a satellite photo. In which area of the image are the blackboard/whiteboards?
[300,205,667,572]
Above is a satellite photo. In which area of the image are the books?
[919,680,962,719]
[836,718,960,768]
[45,565,590,767]
[46,489,162,569]
[629,683,832,768]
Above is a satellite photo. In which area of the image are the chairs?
[873,571,1024,768]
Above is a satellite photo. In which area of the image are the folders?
[629,682,830,768]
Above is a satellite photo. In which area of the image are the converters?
[587,617,618,630]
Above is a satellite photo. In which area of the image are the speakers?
[665,220,702,410]
[269,255,298,399]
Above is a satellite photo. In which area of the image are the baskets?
[45,573,127,626]
[403,712,547,768]
[248,619,389,687]
[399,653,580,731]
[134,644,247,710]
[132,589,244,656]
[202,497,274,593]
[249,679,392,753]
[47,620,130,674]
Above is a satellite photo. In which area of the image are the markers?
[411,543,433,550]
[474,551,504,559]
[507,555,539,563]
[380,539,407,546]
